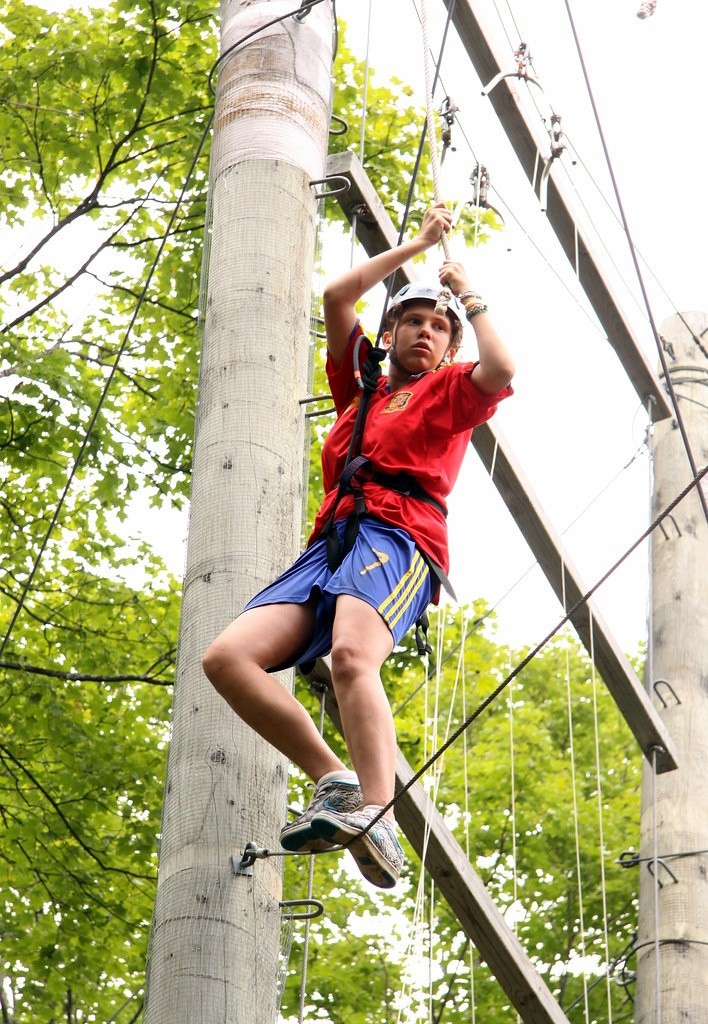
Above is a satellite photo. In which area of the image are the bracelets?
[459,289,488,321]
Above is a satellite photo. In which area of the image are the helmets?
[385,280,464,327]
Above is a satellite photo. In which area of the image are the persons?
[199,200,515,889]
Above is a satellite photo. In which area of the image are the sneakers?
[278,781,364,853]
[311,810,404,888]
